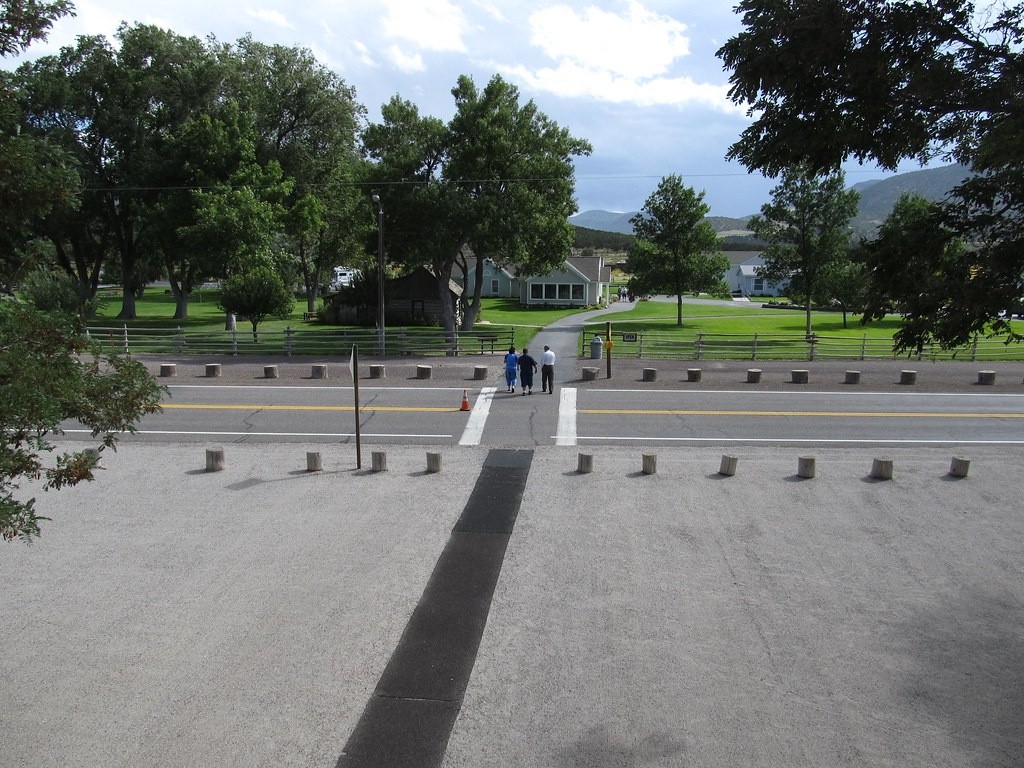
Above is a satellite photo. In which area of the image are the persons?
[629,291,634,297]
[504,347,518,392]
[618,286,627,302]
[518,348,537,396]
[542,346,555,394]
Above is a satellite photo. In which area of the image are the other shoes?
[522,393,525,396]
[549,391,552,394]
[529,391,532,395]
[542,390,546,392]
[508,389,510,391]
[512,388,514,393]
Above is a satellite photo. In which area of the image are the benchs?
[304,312,317,321]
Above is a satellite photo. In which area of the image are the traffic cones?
[459,389,472,411]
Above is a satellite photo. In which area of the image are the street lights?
[371,194,386,357]
